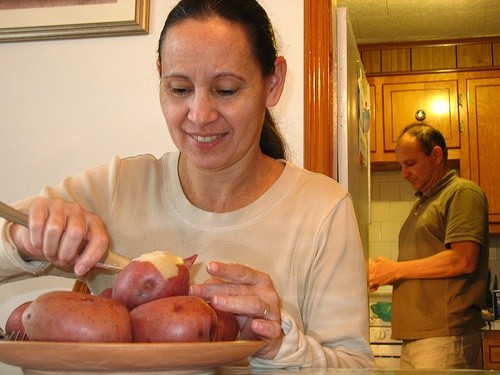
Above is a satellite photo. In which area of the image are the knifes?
[1,201,131,270]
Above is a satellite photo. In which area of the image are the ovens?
[366,284,409,369]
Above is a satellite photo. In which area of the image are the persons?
[0,0,376,370]
[368,122,488,370]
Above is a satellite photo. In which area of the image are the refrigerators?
[335,3,373,308]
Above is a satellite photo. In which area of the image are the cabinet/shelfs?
[370,68,500,235]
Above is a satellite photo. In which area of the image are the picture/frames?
[0,0,152,42]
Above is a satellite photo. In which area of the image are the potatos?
[5,250,240,343]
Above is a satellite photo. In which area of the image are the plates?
[0,330,267,375]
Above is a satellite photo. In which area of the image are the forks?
[0,328,30,342]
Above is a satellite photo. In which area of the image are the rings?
[255,302,267,318]
